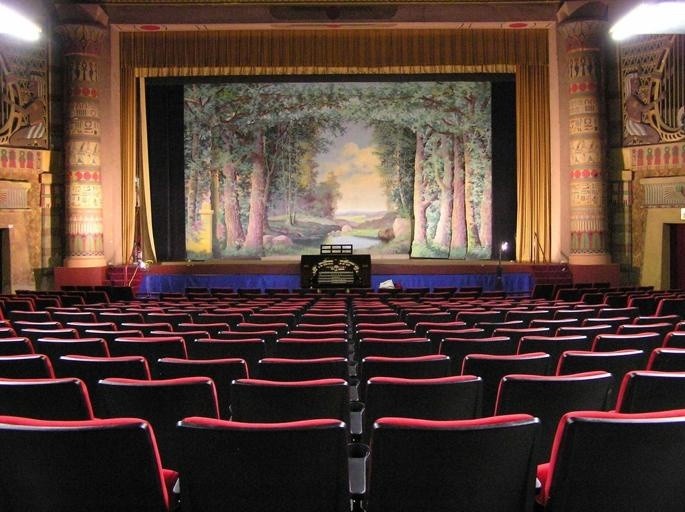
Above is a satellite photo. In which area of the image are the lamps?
[129,258,149,289]
[497,240,509,270]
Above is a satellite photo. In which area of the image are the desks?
[143,272,532,296]
